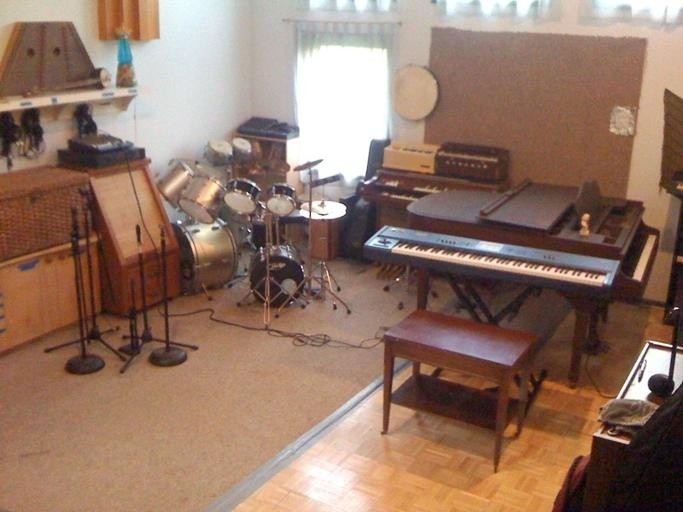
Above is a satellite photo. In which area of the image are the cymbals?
[293,160,322,171]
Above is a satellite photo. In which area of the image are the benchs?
[381,310,537,473]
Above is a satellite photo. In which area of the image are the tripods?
[274,196,351,317]
[43,206,125,362]
[238,218,306,330]
[305,221,341,310]
[120,226,197,372]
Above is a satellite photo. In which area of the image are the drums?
[220,178,260,215]
[179,172,227,224]
[302,199,347,262]
[205,141,234,165]
[153,160,193,208]
[248,245,304,304]
[231,138,252,162]
[265,184,298,217]
[172,218,240,291]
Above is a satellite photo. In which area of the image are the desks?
[584,340,683,511]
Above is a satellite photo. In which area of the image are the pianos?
[362,168,512,233]
[361,226,622,301]
[407,177,660,355]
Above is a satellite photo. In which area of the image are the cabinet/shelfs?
[232,132,304,198]
[1,232,102,355]
